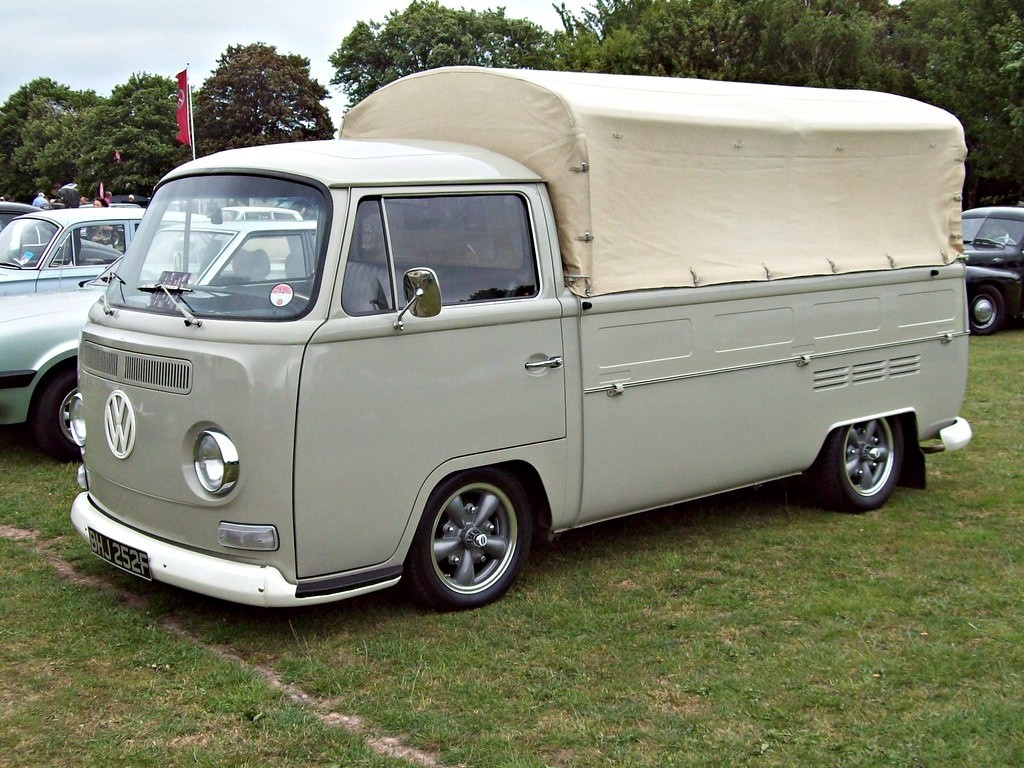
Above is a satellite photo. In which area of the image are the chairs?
[344,260,408,313]
[231,250,304,283]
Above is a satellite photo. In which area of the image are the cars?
[0,201,317,464]
[962,206,1024,336]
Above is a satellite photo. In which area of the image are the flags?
[174,69,190,145]
[113,151,120,162]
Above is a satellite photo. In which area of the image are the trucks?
[69,65,973,612]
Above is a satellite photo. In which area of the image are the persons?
[234,249,271,280]
[32,184,134,248]
